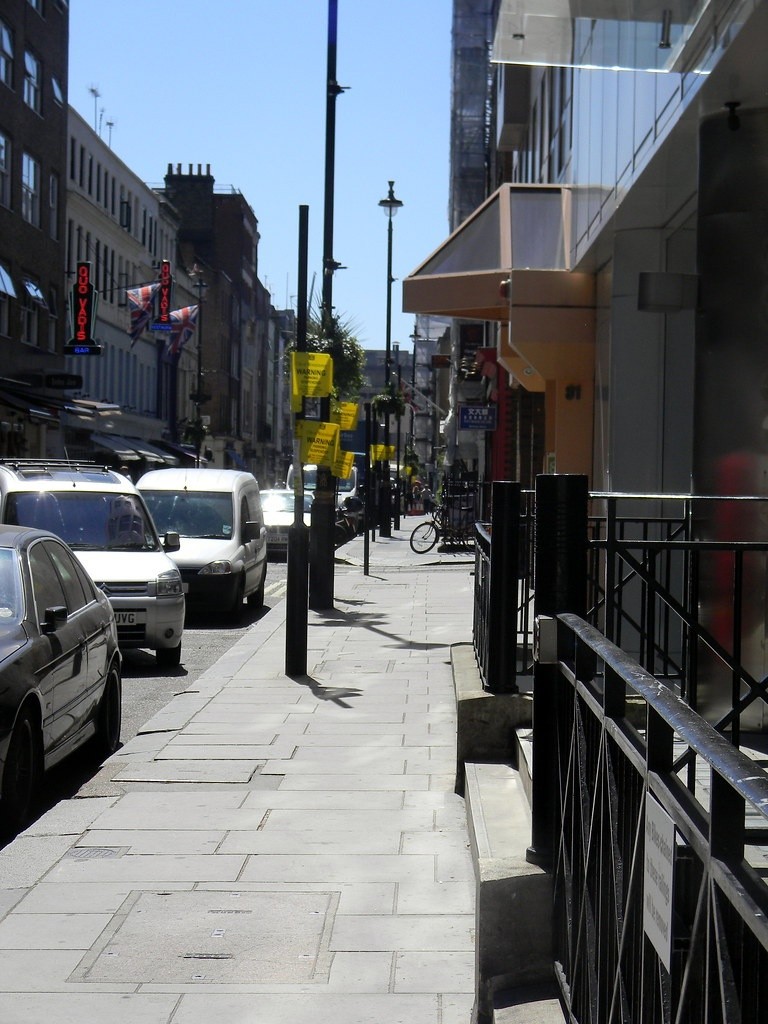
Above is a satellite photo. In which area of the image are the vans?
[283,460,365,534]
[137,468,266,619]
[369,462,405,509]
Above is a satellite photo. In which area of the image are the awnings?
[78,430,208,465]
[224,451,247,469]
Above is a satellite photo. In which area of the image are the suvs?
[0,459,184,672]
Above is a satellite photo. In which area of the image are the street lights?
[194,278,209,472]
[377,182,404,537]
[408,325,423,508]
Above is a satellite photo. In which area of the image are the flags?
[127,283,162,345]
[401,381,423,413]
[166,303,201,355]
[0,388,122,426]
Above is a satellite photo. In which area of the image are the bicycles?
[409,498,478,555]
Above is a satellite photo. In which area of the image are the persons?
[120,466,129,476]
[421,484,432,515]
[412,485,421,510]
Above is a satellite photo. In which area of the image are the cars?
[257,489,318,557]
[2,524,123,821]
